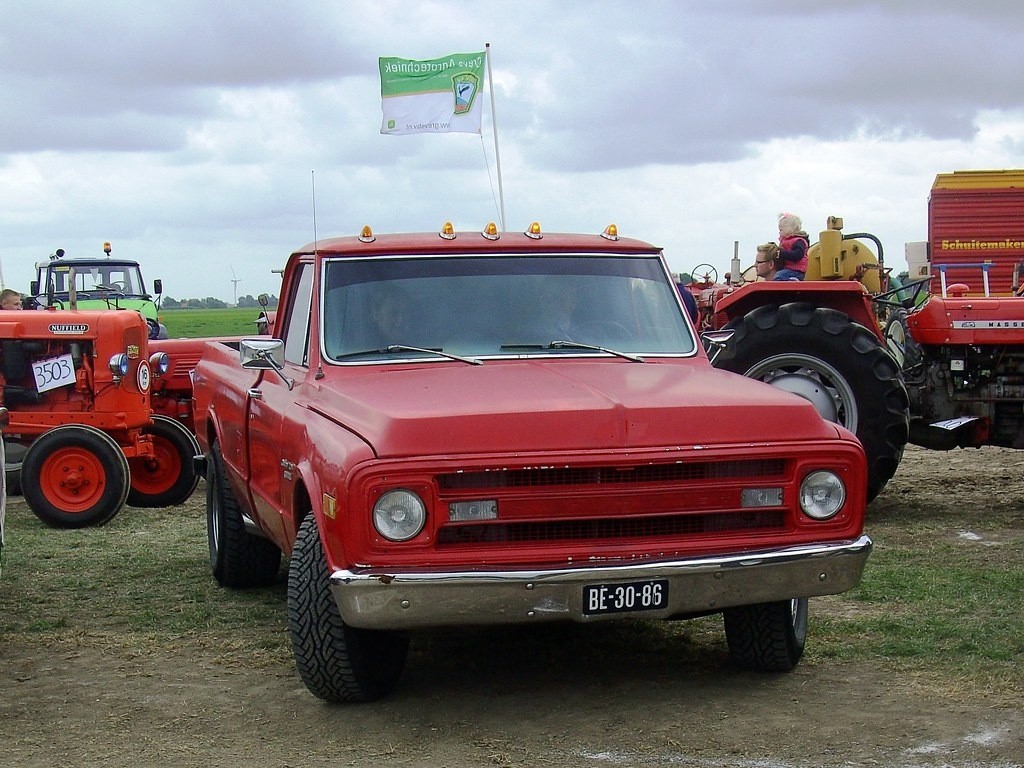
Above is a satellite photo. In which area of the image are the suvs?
[24,242,168,340]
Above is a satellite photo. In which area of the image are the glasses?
[755,260,769,265]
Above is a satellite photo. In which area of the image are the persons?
[773,213,810,281]
[0,289,21,310]
[672,273,697,324]
[541,279,576,332]
[375,288,415,341]
[753,244,784,281]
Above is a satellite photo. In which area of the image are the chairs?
[96,284,121,293]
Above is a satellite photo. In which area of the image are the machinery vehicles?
[3,302,204,532]
[676,241,1024,502]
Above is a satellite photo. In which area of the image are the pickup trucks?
[193,223,876,708]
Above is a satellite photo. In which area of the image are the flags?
[379,51,485,137]
